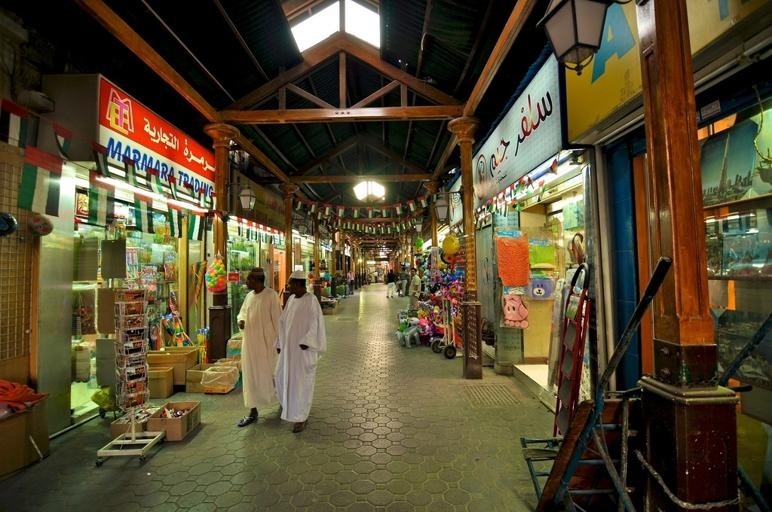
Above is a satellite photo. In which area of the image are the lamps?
[293,214,308,235]
[416,215,431,233]
[353,180,386,204]
[433,186,464,222]
[536,0,650,76]
[226,179,256,211]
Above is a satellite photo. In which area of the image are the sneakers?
[291,422,305,432]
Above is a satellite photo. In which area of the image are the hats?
[290,270,307,280]
[250,268,265,276]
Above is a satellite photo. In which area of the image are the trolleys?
[517,252,772,512]
[431,283,458,359]
[93,286,166,469]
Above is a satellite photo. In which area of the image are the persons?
[236,267,281,427]
[400,267,410,296]
[386,269,396,298]
[275,271,326,433]
[373,271,377,282]
[347,271,355,294]
[409,268,421,309]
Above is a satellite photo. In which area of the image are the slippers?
[237,415,255,427]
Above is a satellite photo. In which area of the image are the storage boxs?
[110,406,160,440]
[147,340,242,399]
[146,401,200,442]
[0,398,51,480]
